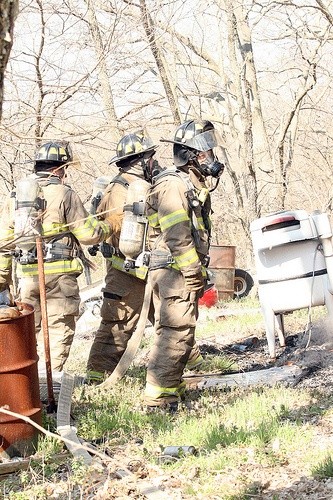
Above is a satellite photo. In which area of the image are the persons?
[0,140,125,408]
[79,131,211,388]
[141,120,218,415]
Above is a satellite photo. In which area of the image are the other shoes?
[178,389,202,402]
[83,378,104,385]
[145,401,190,414]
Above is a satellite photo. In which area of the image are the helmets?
[159,120,218,151]
[107,130,160,165]
[27,143,76,164]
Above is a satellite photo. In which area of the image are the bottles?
[163,446,198,458]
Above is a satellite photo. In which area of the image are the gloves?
[183,271,204,303]
[0,275,11,290]
[106,213,123,232]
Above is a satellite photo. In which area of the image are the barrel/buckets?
[0,302,43,464]
[197,244,237,307]
